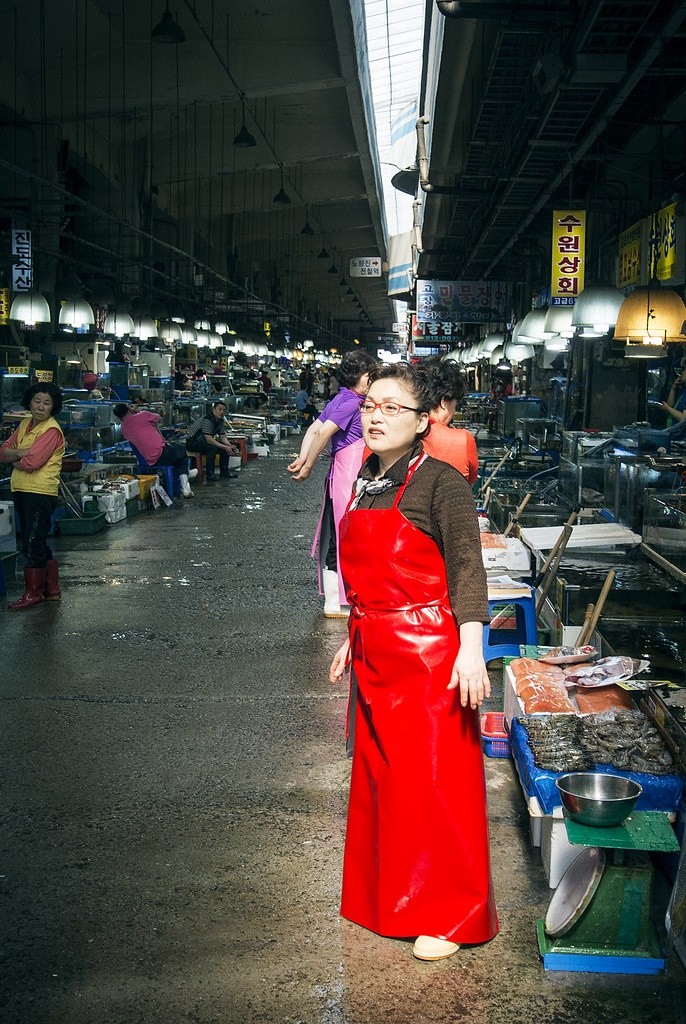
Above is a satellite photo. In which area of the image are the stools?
[226,438,248,464]
[139,465,181,501]
[471,579,536,678]
[185,453,204,480]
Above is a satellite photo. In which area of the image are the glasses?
[682,367,686,371]
[358,399,419,415]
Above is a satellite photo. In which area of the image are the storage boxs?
[475,499,686,890]
[0,374,301,560]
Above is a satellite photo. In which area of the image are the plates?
[538,650,600,664]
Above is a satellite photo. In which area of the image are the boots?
[308,414,313,425]
[8,567,46,610]
[219,456,239,477]
[43,559,61,600]
[179,473,194,498]
[323,564,352,618]
[206,457,220,482]
[188,459,198,480]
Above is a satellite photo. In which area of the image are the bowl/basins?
[60,458,86,472]
[247,452,258,458]
[554,772,643,828]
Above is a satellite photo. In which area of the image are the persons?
[327,363,501,960]
[658,368,686,428]
[0,382,67,610]
[176,369,272,409]
[185,402,239,482]
[113,404,199,498]
[288,350,519,617]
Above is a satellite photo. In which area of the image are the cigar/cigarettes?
[677,373,681,376]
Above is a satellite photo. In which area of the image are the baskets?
[480,712,512,758]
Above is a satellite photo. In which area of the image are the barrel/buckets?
[84,374,98,389]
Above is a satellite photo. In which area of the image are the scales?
[535,807,681,975]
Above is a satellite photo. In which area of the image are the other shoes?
[413,934,461,960]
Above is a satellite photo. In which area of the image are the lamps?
[438,216,686,376]
[147,0,386,344]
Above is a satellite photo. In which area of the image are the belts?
[492,604,540,629]
